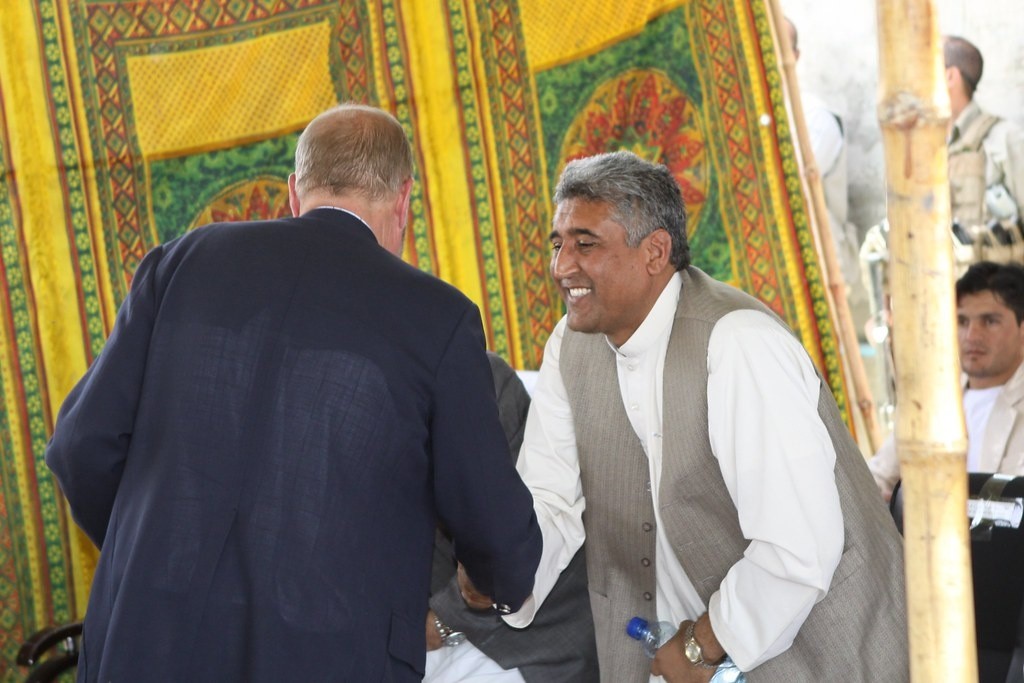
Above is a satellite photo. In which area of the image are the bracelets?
[492,600,511,615]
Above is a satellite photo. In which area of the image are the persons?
[46,105,545,682]
[424,14,1024,683]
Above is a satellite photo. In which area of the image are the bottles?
[627,617,745,683]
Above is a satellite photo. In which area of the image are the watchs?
[434,615,467,647]
[682,623,729,670]
